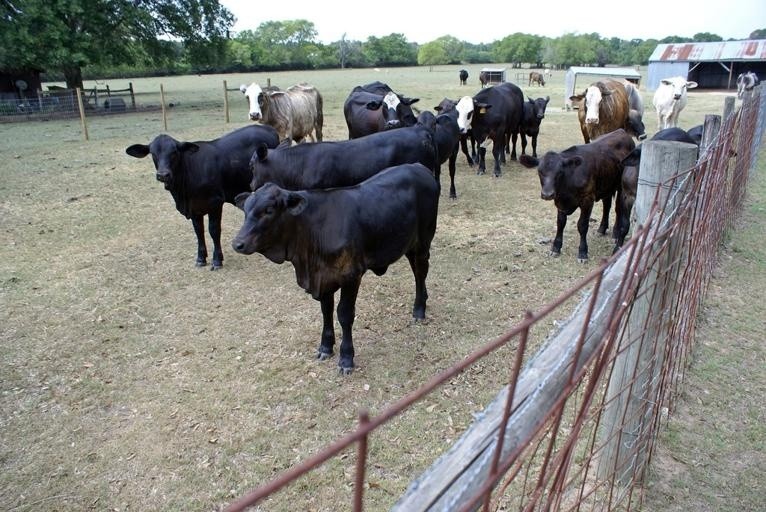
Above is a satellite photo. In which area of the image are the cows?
[238,77,323,145]
[344,81,549,196]
[519,126,703,267]
[614,77,647,142]
[230,123,441,368]
[735,72,759,98]
[652,74,697,132]
[568,73,629,144]
[124,125,281,270]
